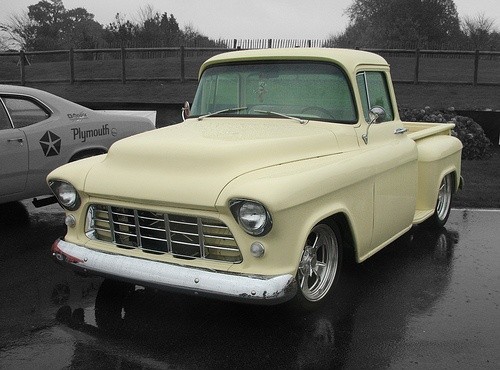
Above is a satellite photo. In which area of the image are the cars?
[43,43,463,316]
[0,83,157,207]
